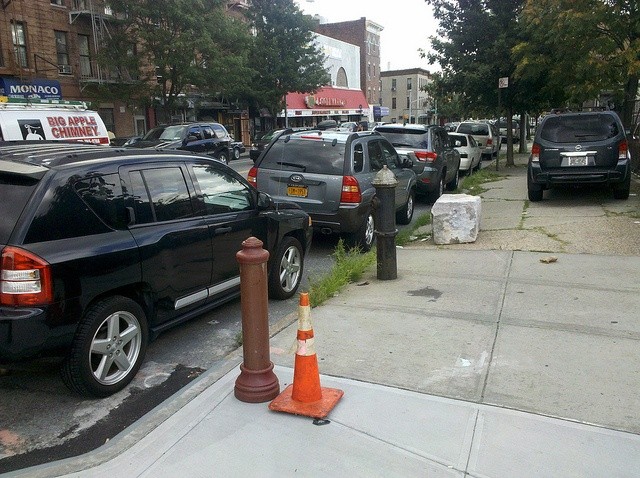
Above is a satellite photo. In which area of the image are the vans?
[0,100,111,142]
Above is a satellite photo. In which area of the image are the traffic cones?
[269,294,345,419]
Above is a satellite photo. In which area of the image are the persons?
[357,124,364,132]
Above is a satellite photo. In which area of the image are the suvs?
[248,128,417,252]
[124,123,234,166]
[456,122,500,160]
[528,106,633,202]
[1,146,312,401]
[372,122,461,198]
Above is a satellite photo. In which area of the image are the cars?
[232,140,246,160]
[448,133,483,178]
[496,121,521,144]
